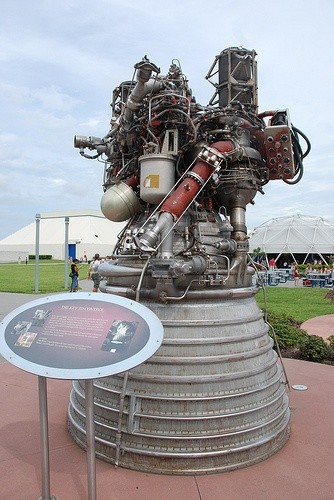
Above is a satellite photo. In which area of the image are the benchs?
[269,274,289,286]
[309,279,334,288]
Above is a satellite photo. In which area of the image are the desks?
[306,273,330,278]
[274,269,291,274]
[267,271,282,285]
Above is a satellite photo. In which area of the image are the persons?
[291,262,297,275]
[294,266,299,286]
[278,257,283,266]
[306,264,312,277]
[87,254,101,292]
[70,259,79,292]
[314,258,317,264]
[269,258,275,272]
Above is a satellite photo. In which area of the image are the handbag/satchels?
[69,273,75,278]
